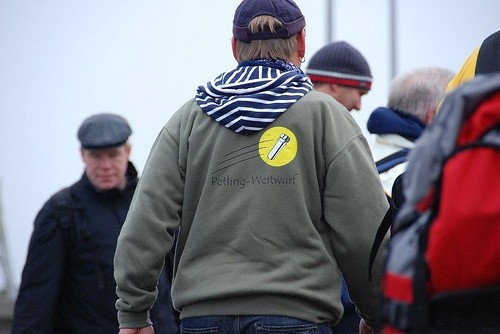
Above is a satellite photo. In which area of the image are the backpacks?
[367,32,500,334]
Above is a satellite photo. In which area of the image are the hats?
[307,41,373,90]
[232,0,305,44]
[78,114,131,149]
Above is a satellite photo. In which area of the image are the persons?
[10,114,180,334]
[305,41,371,334]
[368,67,457,198]
[113,1,390,334]
[382,29,500,334]
